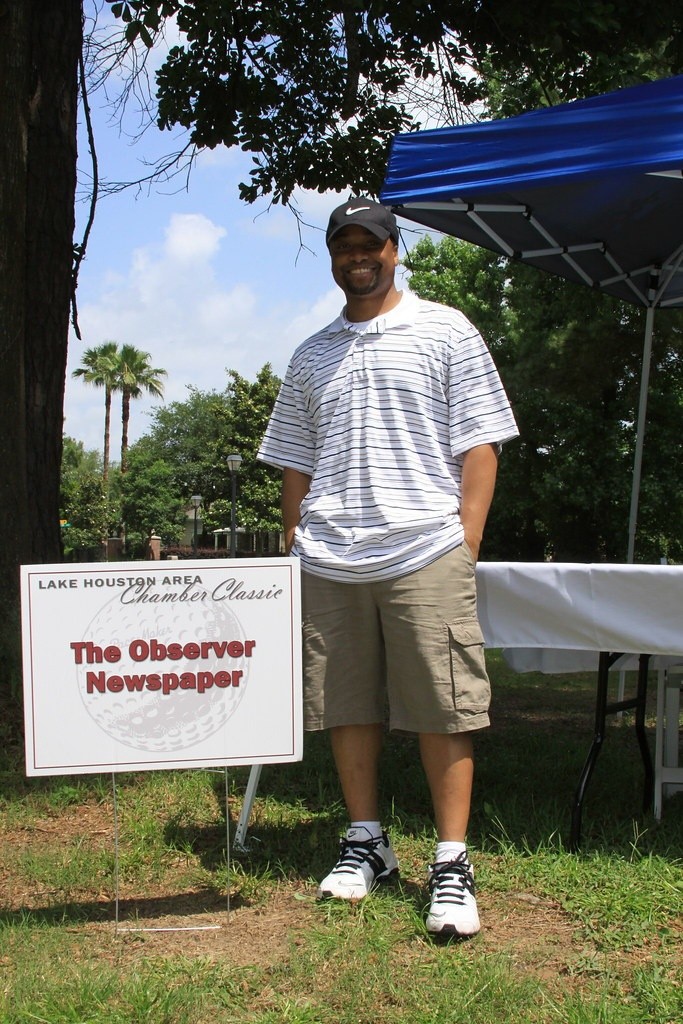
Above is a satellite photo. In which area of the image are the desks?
[228,560,683,865]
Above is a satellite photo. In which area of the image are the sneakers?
[316,826,400,902]
[427,851,480,936]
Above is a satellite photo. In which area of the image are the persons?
[257,196,518,939]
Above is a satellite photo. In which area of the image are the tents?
[231,70,683,853]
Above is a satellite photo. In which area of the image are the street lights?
[225,455,243,558]
[190,495,202,559]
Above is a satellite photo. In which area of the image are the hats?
[325,196,399,250]
